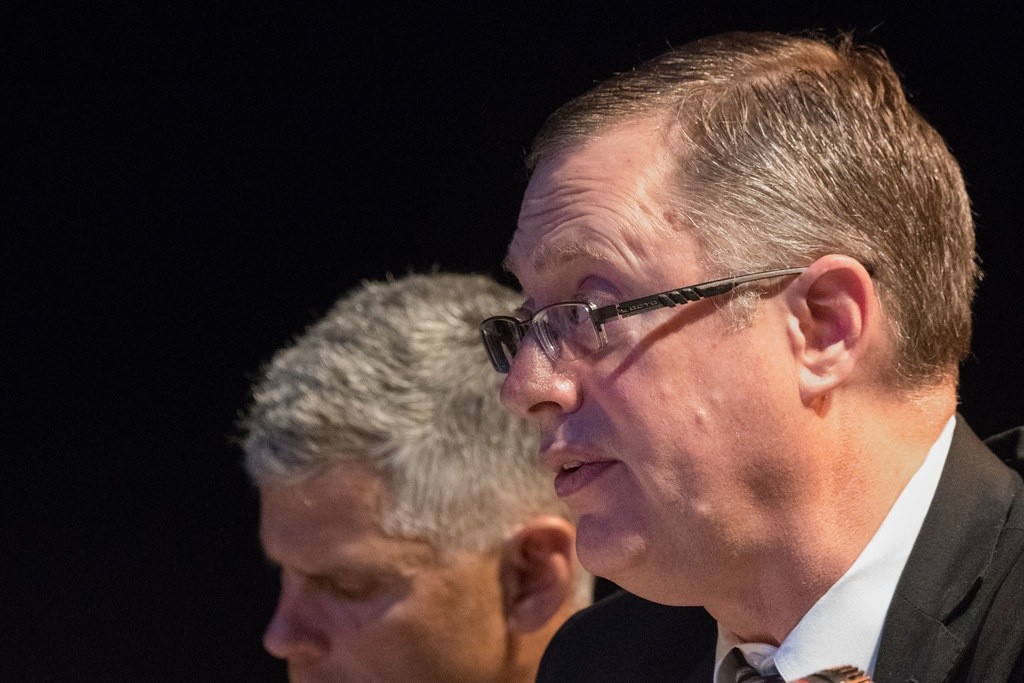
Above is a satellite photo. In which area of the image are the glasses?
[477,262,881,376]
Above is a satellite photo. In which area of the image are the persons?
[496,29,1024,683]
[240,268,591,683]
